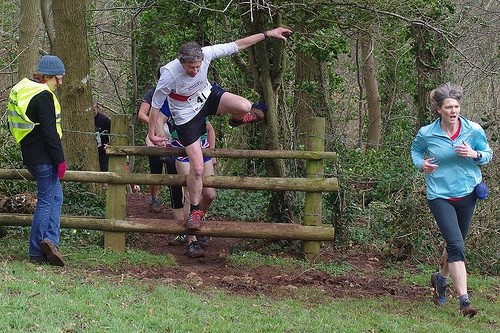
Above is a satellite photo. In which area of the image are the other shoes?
[149,199,160,212]
[196,235,208,246]
[169,234,187,246]
[30,256,48,265]
[40,238,64,266]
[186,241,204,257]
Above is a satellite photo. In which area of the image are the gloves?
[57,160,67,180]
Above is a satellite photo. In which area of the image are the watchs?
[473,151,482,161]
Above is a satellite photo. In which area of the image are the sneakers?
[229,109,264,127]
[187,210,204,231]
[431,272,447,307]
[460,298,477,319]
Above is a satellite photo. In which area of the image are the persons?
[93,100,111,189]
[138,65,216,257]
[8,54,67,267]
[411,81,493,318]
[149,27,292,231]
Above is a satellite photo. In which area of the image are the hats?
[35,55,65,75]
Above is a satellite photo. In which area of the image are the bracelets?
[262,31,268,39]
